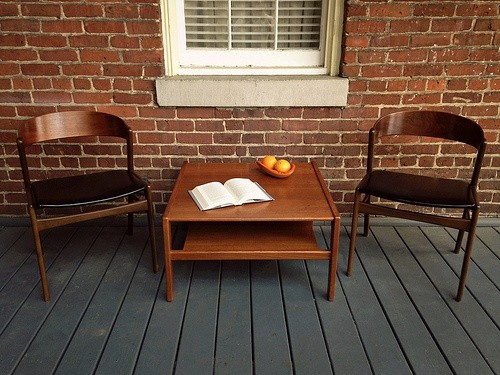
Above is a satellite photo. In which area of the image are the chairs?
[16,111,159,303]
[346,110,487,302]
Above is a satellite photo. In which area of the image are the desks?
[162,160,341,302]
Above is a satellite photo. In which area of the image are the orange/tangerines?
[261,156,278,170]
[274,160,291,173]
